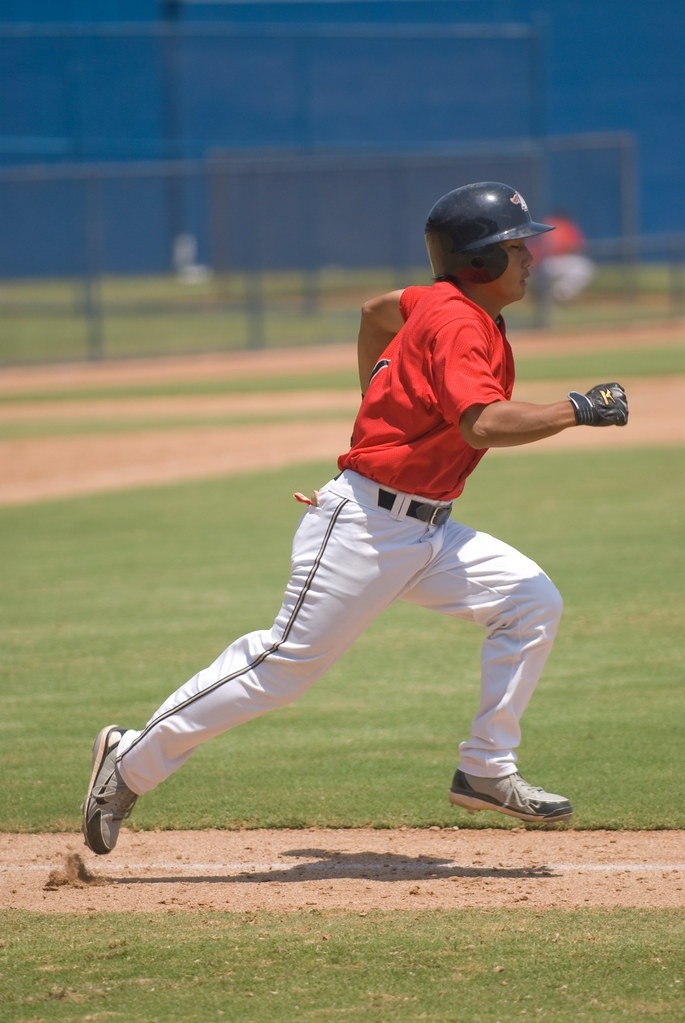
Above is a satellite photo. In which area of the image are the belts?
[334,469,453,526]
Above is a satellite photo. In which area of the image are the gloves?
[569,382,628,426]
[294,490,320,509]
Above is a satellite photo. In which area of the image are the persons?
[81,180,630,856]
[527,218,594,303]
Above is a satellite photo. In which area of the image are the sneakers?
[449,768,572,822]
[80,725,138,855]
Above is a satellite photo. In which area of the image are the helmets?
[425,182,555,282]
